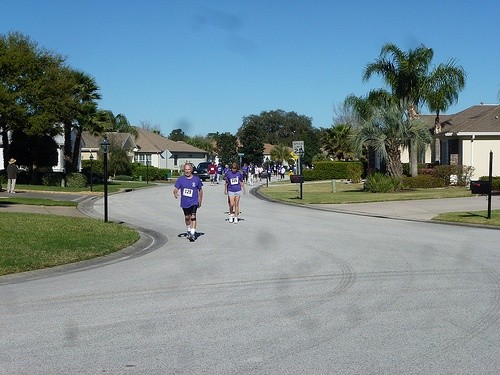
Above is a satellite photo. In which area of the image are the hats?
[8,158,17,164]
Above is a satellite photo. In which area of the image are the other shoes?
[186,230,191,238]
[234,217,238,223]
[229,215,233,222]
[189,234,196,241]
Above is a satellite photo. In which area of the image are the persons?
[173,163,202,242]
[208,161,296,224]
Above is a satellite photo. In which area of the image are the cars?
[193,162,214,182]
